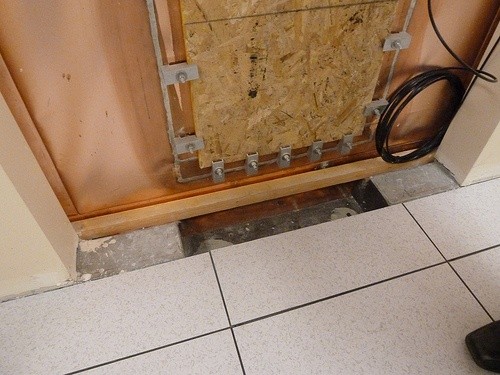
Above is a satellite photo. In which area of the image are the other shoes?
[466,320,500,374]
